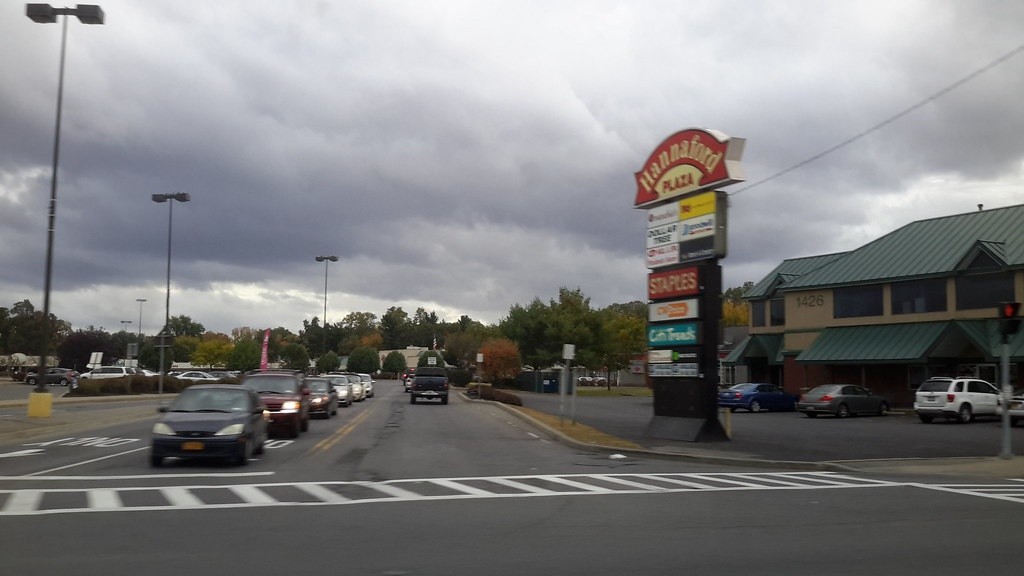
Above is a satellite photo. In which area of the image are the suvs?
[913,377,1004,423]
[237,369,312,439]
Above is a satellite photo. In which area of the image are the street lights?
[120,320,132,359]
[136,299,146,367]
[151,193,190,405]
[25,3,105,420]
[315,255,339,376]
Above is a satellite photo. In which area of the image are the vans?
[80,366,145,380]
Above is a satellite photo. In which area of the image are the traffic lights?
[1000,302,1021,335]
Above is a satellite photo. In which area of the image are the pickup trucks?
[410,367,449,405]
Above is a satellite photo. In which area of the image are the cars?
[402,367,416,386]
[995,393,1024,426]
[405,374,415,392]
[320,375,354,407]
[335,375,367,402]
[357,374,376,398]
[795,385,891,419]
[26,368,80,386]
[148,385,270,467]
[304,378,338,419]
[142,370,161,376]
[166,371,243,381]
[12,365,49,381]
[717,383,801,413]
